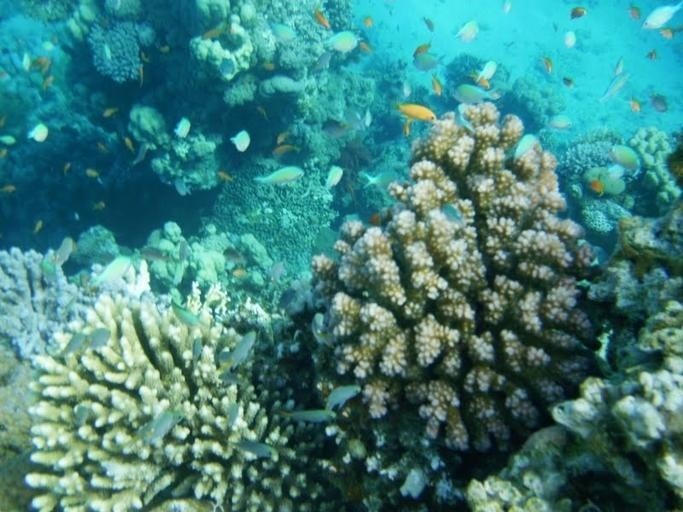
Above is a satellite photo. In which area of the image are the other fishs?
[0,0,683,372]
